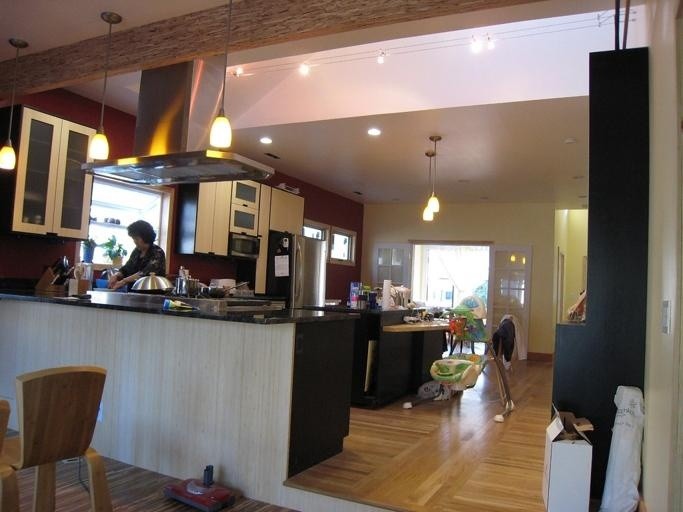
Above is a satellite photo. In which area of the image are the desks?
[382,316,450,394]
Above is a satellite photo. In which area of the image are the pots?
[131,272,174,296]
[200,282,249,298]
[176,275,199,298]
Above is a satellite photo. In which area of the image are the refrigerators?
[264,309,325,323]
[266,235,327,308]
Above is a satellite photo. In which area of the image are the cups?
[358,295,366,311]
[369,293,377,308]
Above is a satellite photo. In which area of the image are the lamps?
[206,2,235,151]
[0,38,29,171]
[87,11,122,163]
[420,152,437,224]
[427,135,442,214]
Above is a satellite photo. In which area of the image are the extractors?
[80,53,275,187]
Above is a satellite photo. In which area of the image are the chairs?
[0,365,114,511]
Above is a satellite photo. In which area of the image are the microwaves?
[229,232,260,260]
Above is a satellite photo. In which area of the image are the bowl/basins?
[96,279,109,288]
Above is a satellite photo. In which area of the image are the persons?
[108,219,165,294]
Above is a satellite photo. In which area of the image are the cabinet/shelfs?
[194,181,261,254]
[7,106,96,237]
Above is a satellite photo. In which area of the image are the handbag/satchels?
[567,289,587,322]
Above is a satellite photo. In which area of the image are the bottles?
[350,282,359,309]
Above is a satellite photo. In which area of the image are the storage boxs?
[540,400,593,512]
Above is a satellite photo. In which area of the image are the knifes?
[51,256,75,285]
